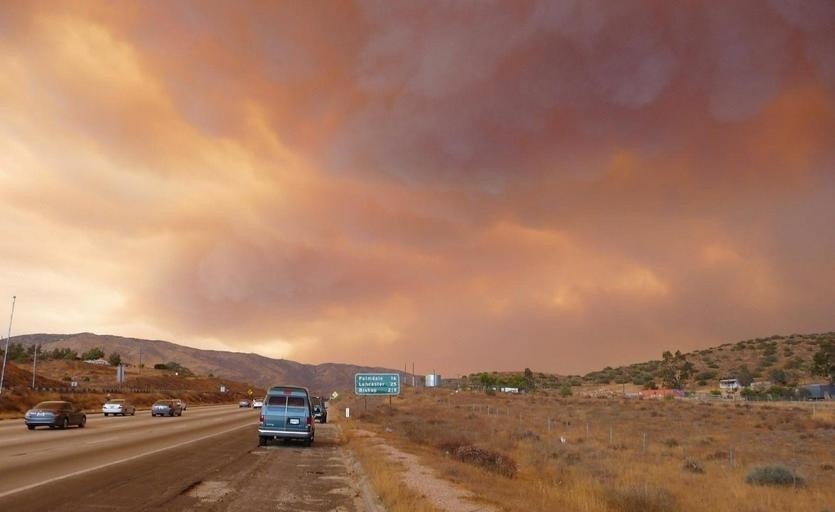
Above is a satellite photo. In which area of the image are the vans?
[258,385,315,446]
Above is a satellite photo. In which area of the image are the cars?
[151,398,187,417]
[314,396,327,423]
[101,398,136,416]
[239,397,264,409]
[24,400,87,430]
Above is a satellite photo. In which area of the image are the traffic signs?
[355,374,399,396]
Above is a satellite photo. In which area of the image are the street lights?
[0,295,16,394]
